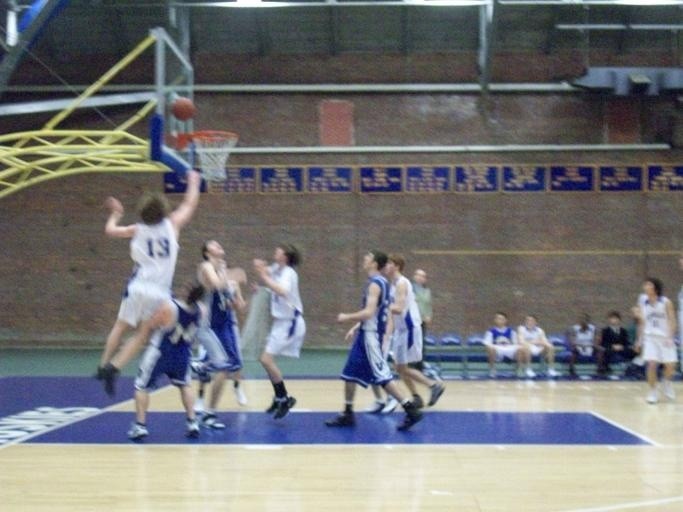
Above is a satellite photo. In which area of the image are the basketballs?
[170,100,194,119]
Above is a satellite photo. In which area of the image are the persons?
[630,257,683,405]
[565,312,606,381]
[517,314,559,379]
[89,171,246,442]
[600,312,638,380]
[481,311,525,379]
[325,248,446,431]
[249,243,307,418]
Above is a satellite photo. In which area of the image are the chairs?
[424,334,639,381]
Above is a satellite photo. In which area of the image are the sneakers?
[596,366,613,380]
[409,393,425,408]
[94,367,109,381]
[325,412,356,428]
[661,379,676,401]
[396,407,425,432]
[381,394,401,416]
[200,410,227,431]
[184,417,201,440]
[127,421,150,440]
[100,364,117,397]
[265,397,282,415]
[646,386,661,404]
[624,366,640,382]
[544,366,562,379]
[524,367,537,379]
[427,380,448,407]
[515,365,526,379]
[234,384,248,407]
[488,365,497,380]
[193,396,206,415]
[568,368,580,380]
[272,395,296,420]
[366,399,385,414]
